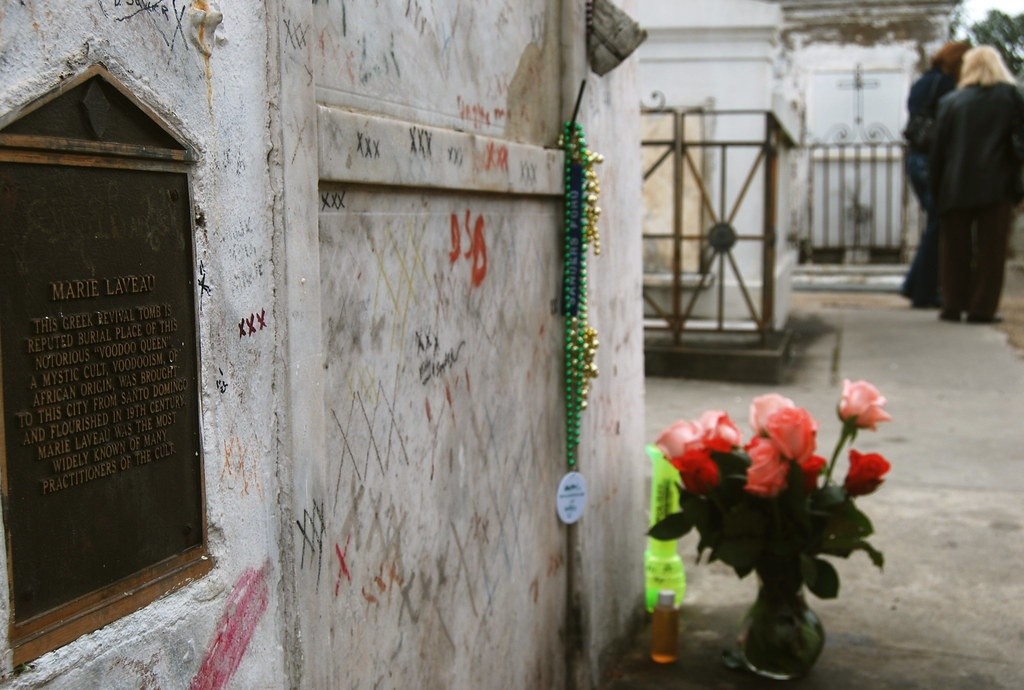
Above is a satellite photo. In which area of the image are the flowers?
[640,379,915,603]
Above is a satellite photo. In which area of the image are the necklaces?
[555,120,588,524]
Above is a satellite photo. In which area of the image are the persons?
[898,40,973,308]
[918,45,1024,322]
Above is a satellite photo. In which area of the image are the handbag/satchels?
[904,115,937,150]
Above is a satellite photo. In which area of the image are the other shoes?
[940,308,961,321]
[967,312,1002,323]
[901,282,940,308]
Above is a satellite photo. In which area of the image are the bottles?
[644,442,686,611]
[648,588,681,663]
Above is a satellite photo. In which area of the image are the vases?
[741,560,830,681]
